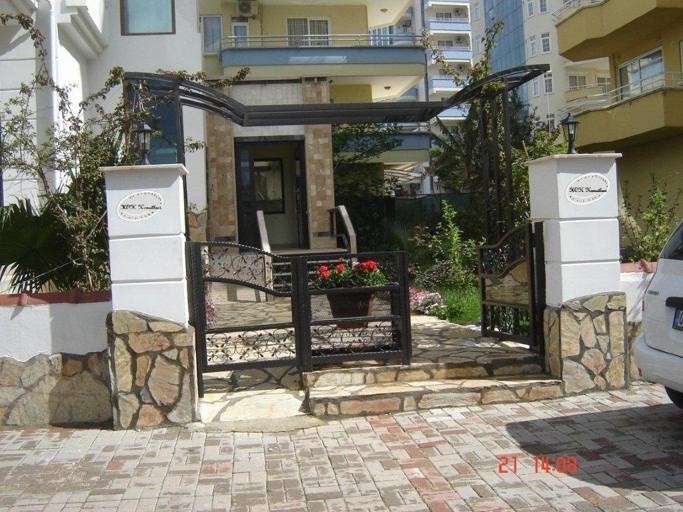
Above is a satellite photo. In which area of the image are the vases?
[325,288,373,329]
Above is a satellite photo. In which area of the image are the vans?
[631,222,683,409]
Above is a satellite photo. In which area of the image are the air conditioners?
[234,2,258,19]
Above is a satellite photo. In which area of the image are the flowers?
[407,286,442,317]
[314,258,386,290]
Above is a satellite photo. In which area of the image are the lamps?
[132,122,152,165]
[560,113,581,154]
